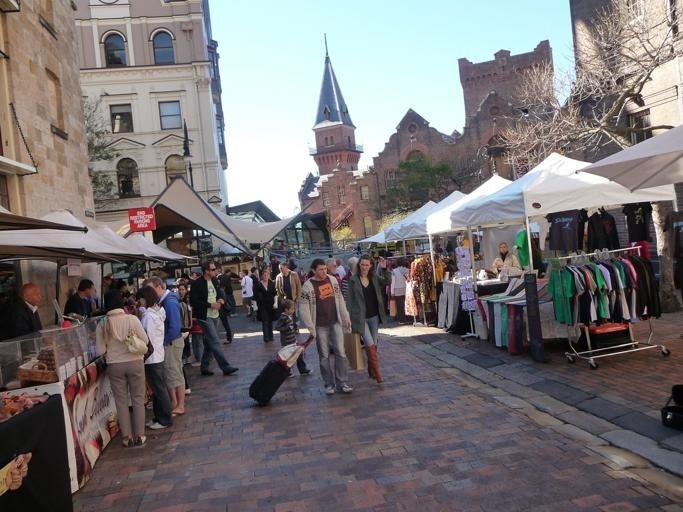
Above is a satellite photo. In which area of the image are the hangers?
[554,248,639,270]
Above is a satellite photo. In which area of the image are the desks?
[0,394,71,500]
[446,281,581,345]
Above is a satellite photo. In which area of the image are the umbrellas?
[0,203,199,325]
[568,122,682,193]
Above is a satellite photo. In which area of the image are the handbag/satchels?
[276,343,305,369]
[126,335,148,355]
[344,327,365,370]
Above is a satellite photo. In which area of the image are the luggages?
[250,333,314,404]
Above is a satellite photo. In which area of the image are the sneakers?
[122,366,238,451]
[286,370,352,394]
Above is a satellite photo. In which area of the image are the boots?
[365,344,383,383]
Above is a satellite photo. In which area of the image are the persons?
[94,290,154,448]
[239,254,392,395]
[510,228,531,272]
[391,253,459,324]
[1,259,242,430]
[489,242,520,278]
